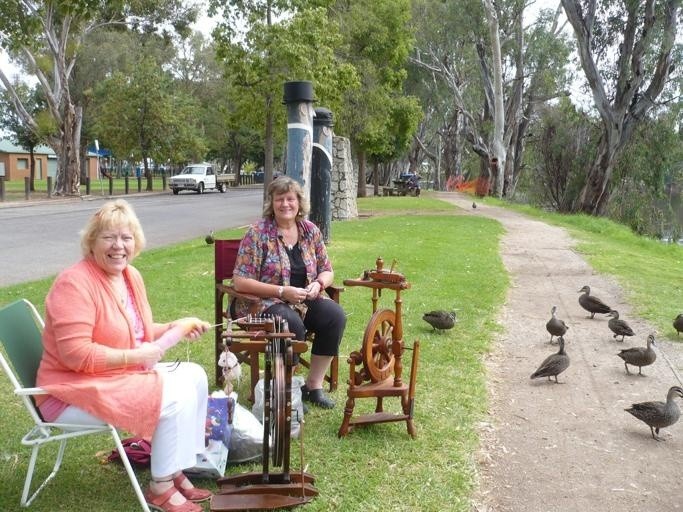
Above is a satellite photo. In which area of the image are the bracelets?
[278,285,285,298]
[315,278,325,291]
[121,350,129,368]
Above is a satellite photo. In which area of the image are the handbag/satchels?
[183,397,235,479]
[107,436,151,466]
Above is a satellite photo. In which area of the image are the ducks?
[422,310,456,335]
[530,337,570,384]
[617,335,657,377]
[205,230,214,244]
[578,286,612,319]
[673,314,683,336]
[472,202,477,208]
[624,386,683,442]
[546,306,570,344]
[605,310,636,342]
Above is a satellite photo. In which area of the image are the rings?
[298,300,301,304]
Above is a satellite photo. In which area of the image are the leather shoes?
[145,483,204,512]
[174,474,214,503]
[301,385,335,411]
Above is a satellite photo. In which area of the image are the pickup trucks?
[166,162,236,194]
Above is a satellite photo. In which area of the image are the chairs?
[214,237,345,403]
[0,298,152,511]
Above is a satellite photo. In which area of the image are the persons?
[228,174,347,409]
[33,199,213,512]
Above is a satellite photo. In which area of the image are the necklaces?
[106,275,124,304]
